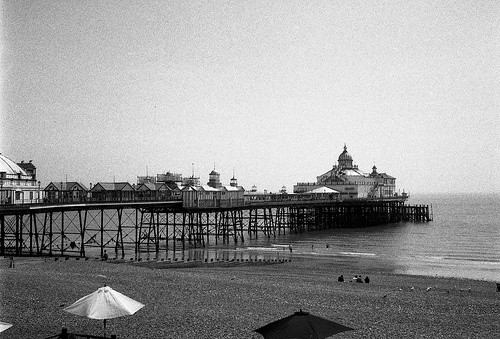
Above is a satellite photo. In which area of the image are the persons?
[338,275,370,283]
[102,250,108,261]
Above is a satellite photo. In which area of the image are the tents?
[306,186,339,199]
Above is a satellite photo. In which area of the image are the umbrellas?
[62,283,145,339]
